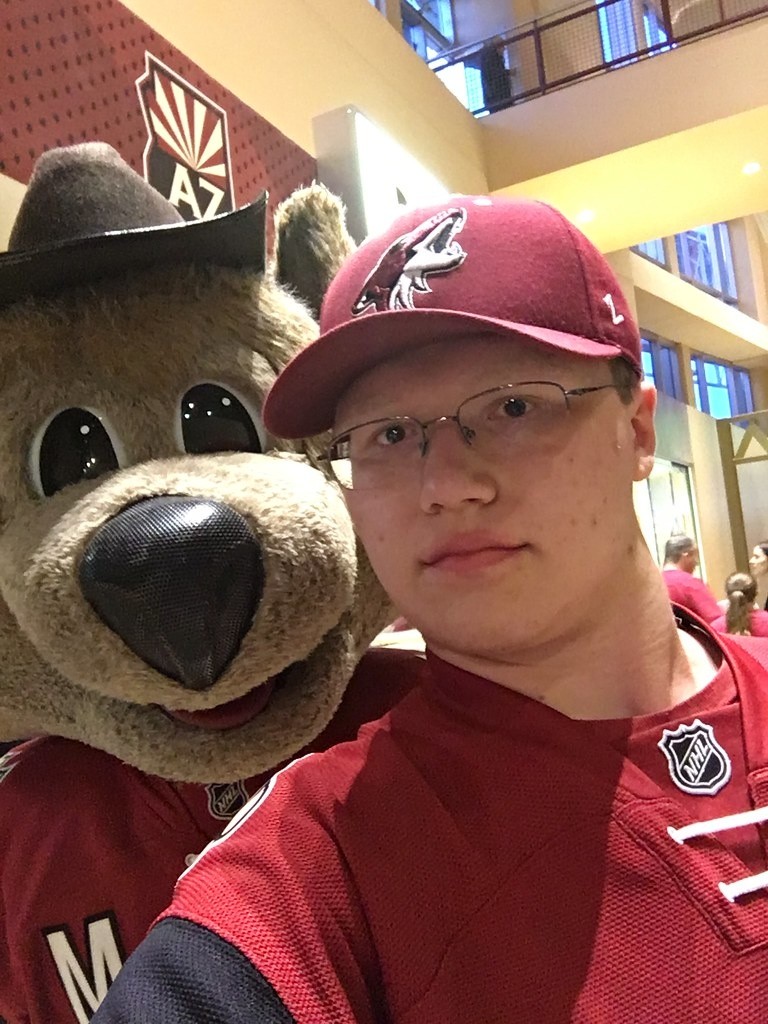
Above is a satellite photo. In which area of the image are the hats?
[263,195,643,439]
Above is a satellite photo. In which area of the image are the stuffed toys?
[0,140,427,1023]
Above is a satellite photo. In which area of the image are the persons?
[91,192,767,1022]
[660,534,768,641]
[481,36,517,115]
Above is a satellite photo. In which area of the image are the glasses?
[317,381,617,491]
